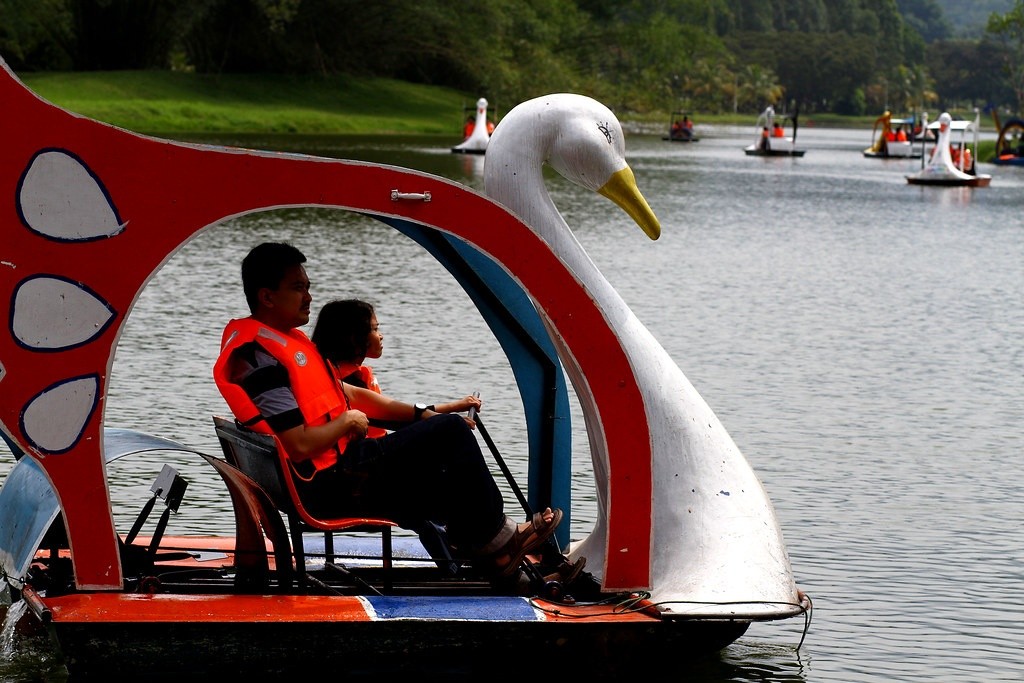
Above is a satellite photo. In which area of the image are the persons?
[672,116,693,129]
[762,126,769,137]
[931,143,973,172]
[310,299,586,593]
[771,122,784,137]
[889,120,927,142]
[213,241,564,581]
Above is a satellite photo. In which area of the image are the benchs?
[212,415,398,582]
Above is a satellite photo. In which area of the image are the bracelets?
[427,405,435,412]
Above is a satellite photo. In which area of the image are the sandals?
[491,507,563,577]
[543,556,587,593]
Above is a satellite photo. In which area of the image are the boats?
[986,118,1024,166]
[861,110,925,162]
[743,104,808,158]
[662,110,702,141]
[0,55,813,681]
[900,108,994,188]
[906,111,937,141]
[449,98,498,155]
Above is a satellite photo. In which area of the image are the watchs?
[414,403,427,417]
